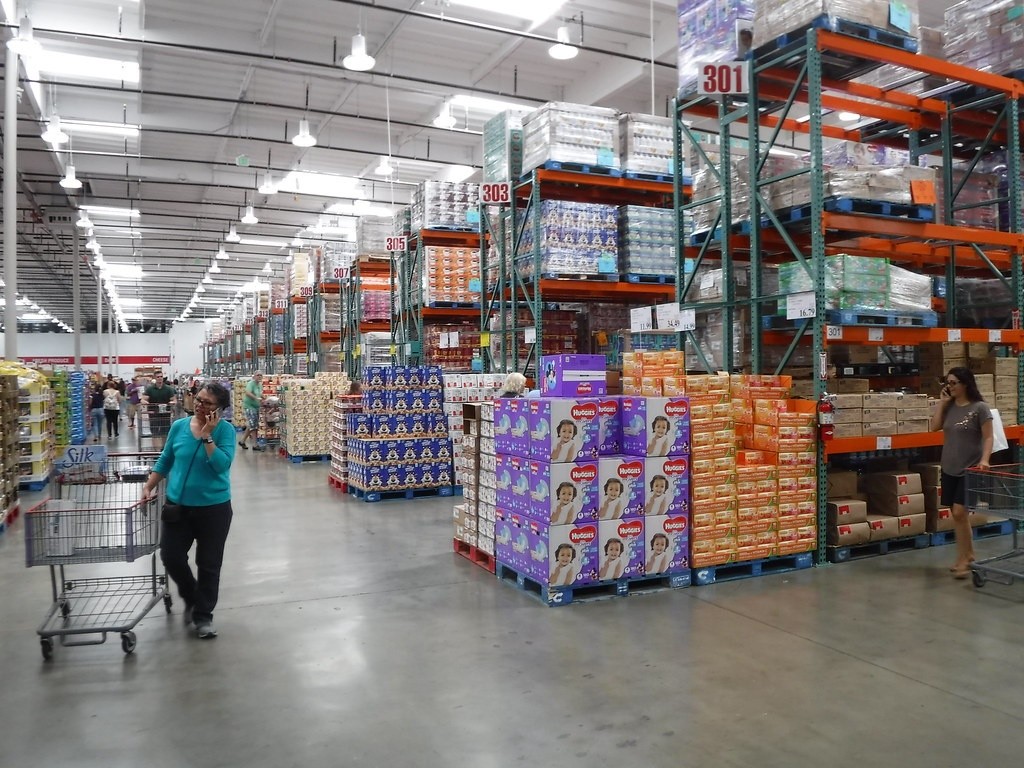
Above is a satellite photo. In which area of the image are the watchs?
[202,436,213,443]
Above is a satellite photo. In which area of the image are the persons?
[165,379,178,396]
[238,370,264,450]
[550,416,671,586]
[931,367,993,577]
[140,382,237,637]
[191,380,202,403]
[740,29,752,50]
[142,370,176,452]
[854,143,897,164]
[89,374,145,440]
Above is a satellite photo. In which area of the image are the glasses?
[156,376,163,378]
[193,395,218,408]
[945,379,963,388]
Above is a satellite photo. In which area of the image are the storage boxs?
[348,1,1024,587]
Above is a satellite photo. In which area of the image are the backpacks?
[103,389,119,409]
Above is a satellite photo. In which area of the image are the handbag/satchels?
[161,503,183,523]
[990,409,1009,455]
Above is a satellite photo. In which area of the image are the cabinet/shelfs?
[479,168,692,389]
[0,369,101,532]
[207,28,1024,564]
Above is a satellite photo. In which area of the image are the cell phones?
[945,389,950,396]
[208,407,224,421]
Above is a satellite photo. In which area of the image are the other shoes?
[253,445,265,452]
[184,604,195,624]
[238,441,249,449]
[950,555,976,579]
[115,434,119,438]
[196,621,217,638]
[93,438,98,442]
[108,437,112,441]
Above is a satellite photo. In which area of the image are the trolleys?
[259,399,283,451]
[136,401,176,466]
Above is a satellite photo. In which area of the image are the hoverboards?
[25,452,173,659]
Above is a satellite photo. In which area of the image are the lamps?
[374,157,395,175]
[290,110,317,148]
[291,230,303,247]
[176,191,272,322]
[7,4,46,57]
[342,0,376,71]
[547,2,578,60]
[434,86,457,128]
[256,170,279,196]
[3,82,131,333]
